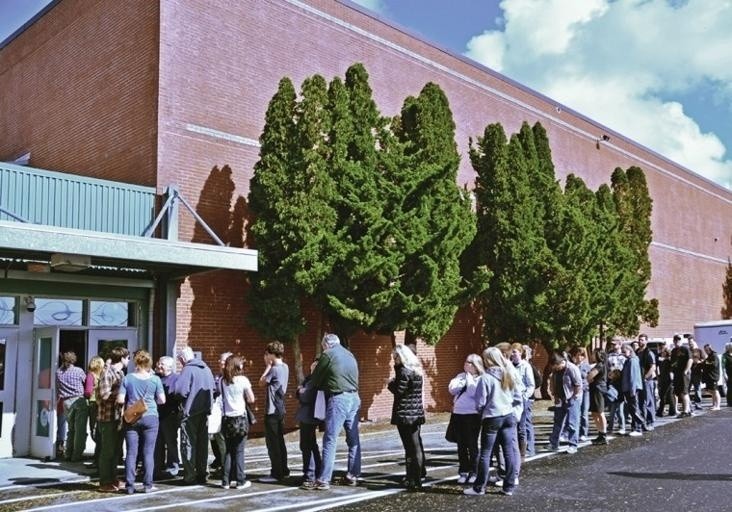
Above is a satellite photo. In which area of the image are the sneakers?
[677,412,687,418]
[114,481,125,488]
[566,447,577,454]
[236,481,251,489]
[458,472,470,483]
[501,490,512,496]
[464,487,484,495]
[468,473,477,483]
[544,444,556,451]
[496,479,518,486]
[629,431,642,436]
[98,485,118,491]
[617,428,625,434]
[687,413,691,416]
[333,475,357,486]
[489,477,499,482]
[258,476,279,482]
[144,487,158,492]
[303,481,329,490]
[221,485,229,489]
[271,469,290,479]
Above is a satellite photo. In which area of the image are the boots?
[591,431,606,443]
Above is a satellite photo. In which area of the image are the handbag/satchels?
[207,396,224,434]
[445,414,457,443]
[158,462,179,478]
[606,384,619,402]
[124,400,147,425]
[314,391,326,420]
[222,416,249,439]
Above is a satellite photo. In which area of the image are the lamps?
[596,133,611,144]
[51,252,93,275]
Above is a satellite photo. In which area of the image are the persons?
[384,343,427,488]
[88,419,100,476]
[257,339,291,482]
[38,350,67,457]
[211,351,233,476]
[97,346,131,492]
[542,333,732,456]
[299,333,362,490]
[295,357,323,485]
[445,341,542,495]
[159,356,181,478]
[176,346,218,483]
[117,351,166,494]
[56,351,91,465]
[219,355,255,490]
[102,357,111,370]
[84,355,106,459]
[131,349,142,359]
[154,359,160,376]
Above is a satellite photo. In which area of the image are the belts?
[63,395,78,400]
[334,390,356,396]
